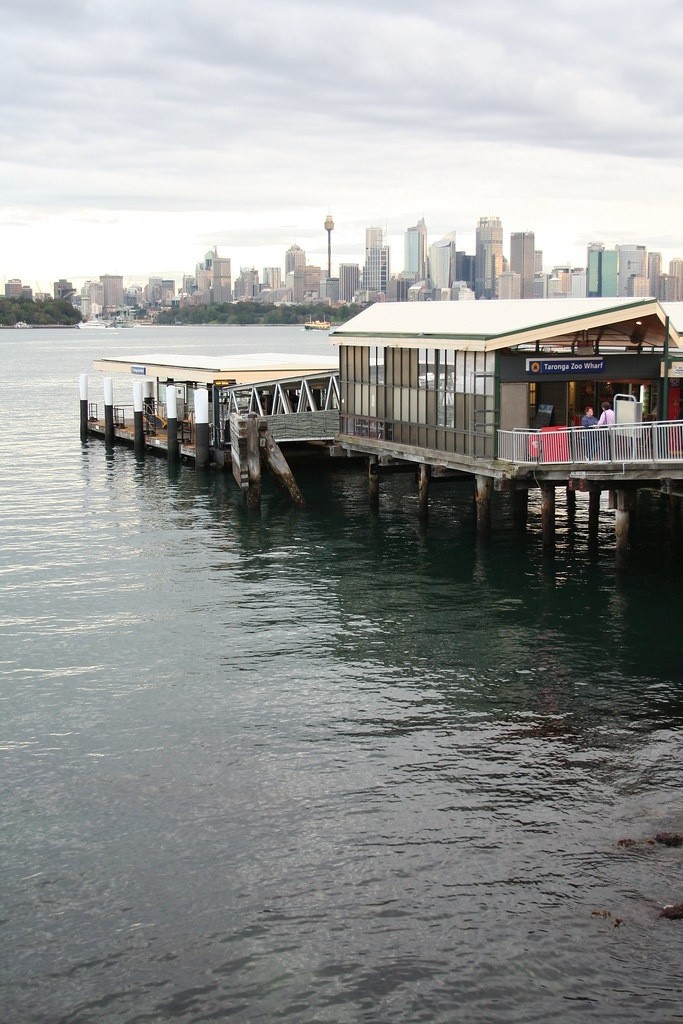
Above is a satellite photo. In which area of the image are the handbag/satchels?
[600,426,608,436]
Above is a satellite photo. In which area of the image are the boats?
[76,320,106,329]
[13,321,34,329]
[110,313,135,329]
[304,320,331,331]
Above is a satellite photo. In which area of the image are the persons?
[581,406,601,460]
[596,401,615,460]
